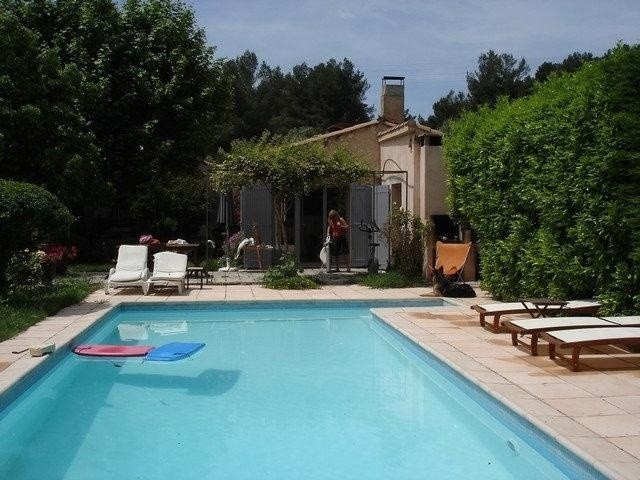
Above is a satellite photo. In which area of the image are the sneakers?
[335,265,352,274]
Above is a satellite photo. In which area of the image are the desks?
[518,297,567,339]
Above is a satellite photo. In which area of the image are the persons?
[325,208,353,272]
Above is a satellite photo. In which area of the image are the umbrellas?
[215,192,238,273]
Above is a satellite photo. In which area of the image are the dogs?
[420,265,477,298]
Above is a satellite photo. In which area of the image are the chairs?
[501,314,640,356]
[428,240,472,285]
[118,321,148,344]
[470,300,604,333]
[149,319,192,336]
[539,326,639,371]
[104,236,297,296]
